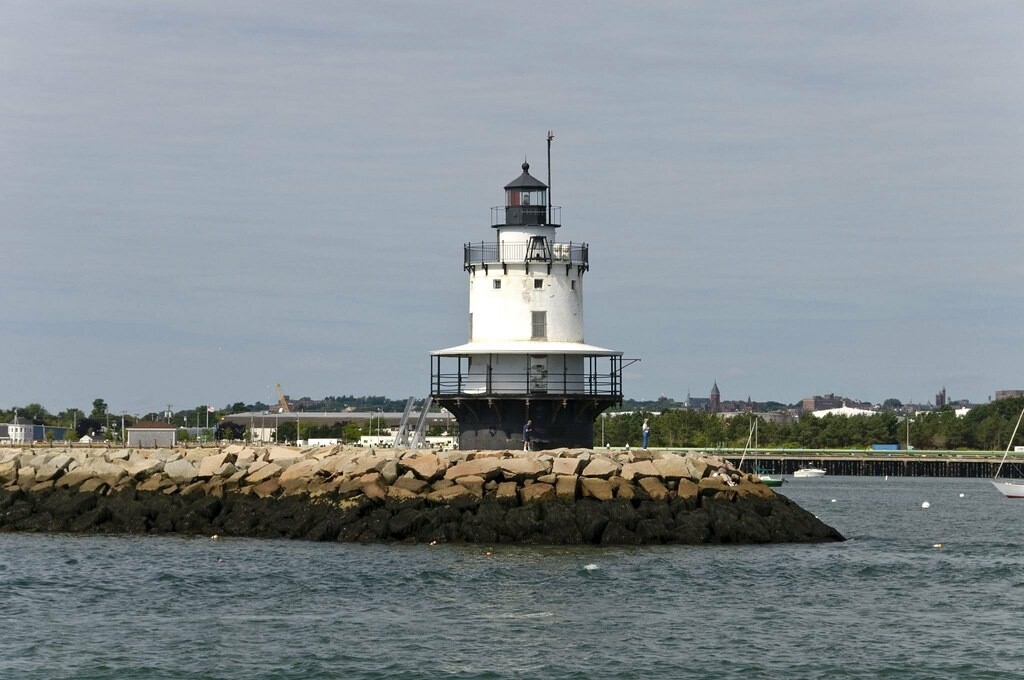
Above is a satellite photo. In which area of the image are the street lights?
[902,413,910,449]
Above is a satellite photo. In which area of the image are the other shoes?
[642,448,647,451]
[524,449,527,452]
[528,450,533,452]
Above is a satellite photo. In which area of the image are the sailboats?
[989,407,1024,498]
[736,417,789,487]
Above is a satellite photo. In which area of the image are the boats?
[793,461,827,478]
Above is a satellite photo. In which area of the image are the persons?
[523,420,533,451]
[642,417,650,449]
